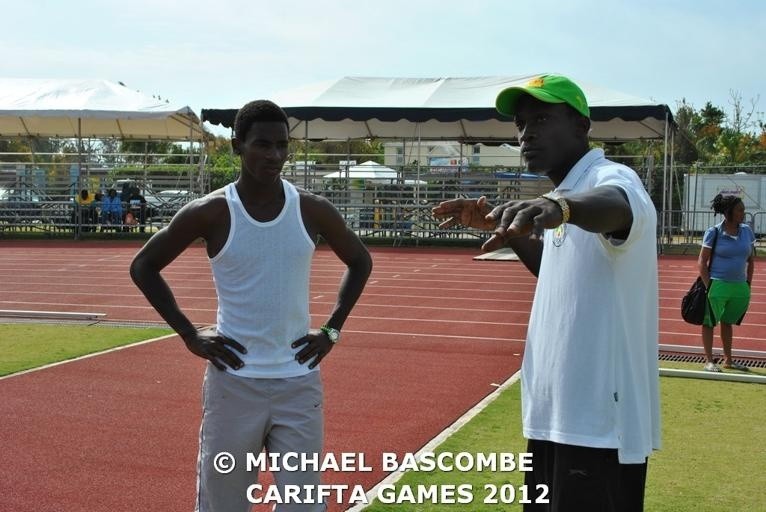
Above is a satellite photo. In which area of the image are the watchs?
[320,326,340,344]
[538,193,570,224]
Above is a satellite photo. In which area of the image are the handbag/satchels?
[680,276,706,326]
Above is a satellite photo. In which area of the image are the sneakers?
[723,362,747,370]
[704,361,720,372]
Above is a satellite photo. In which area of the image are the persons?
[129,100,373,512]
[74,188,147,234]
[431,73,662,512]
[698,194,757,372]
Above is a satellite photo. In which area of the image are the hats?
[496,74,590,120]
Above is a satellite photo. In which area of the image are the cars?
[0,177,198,229]
